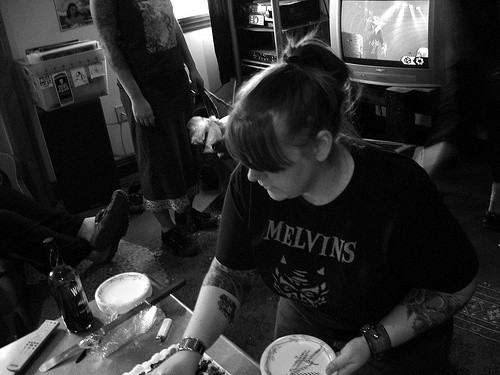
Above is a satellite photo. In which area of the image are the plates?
[260,335,339,375]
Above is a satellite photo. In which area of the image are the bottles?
[43,237,95,335]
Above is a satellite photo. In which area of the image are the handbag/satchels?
[188,84,221,188]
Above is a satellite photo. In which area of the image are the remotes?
[7,319,59,373]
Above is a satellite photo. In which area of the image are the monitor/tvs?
[328,0,461,89]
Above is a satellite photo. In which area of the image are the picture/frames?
[53,0,93,31]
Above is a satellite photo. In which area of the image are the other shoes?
[89,189,130,253]
[98,216,129,265]
[483,212,500,231]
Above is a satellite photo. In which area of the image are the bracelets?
[177,336,207,355]
[361,320,391,361]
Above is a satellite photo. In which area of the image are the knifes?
[75,341,100,363]
[39,276,187,371]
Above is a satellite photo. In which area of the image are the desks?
[0,276,263,375]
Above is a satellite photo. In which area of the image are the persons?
[145,31,480,375]
[65,3,84,26]
[87,0,218,257]
[0,170,130,272]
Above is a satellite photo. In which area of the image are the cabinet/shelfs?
[241,0,327,69]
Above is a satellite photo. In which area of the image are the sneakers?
[161,226,199,256]
[174,205,220,233]
